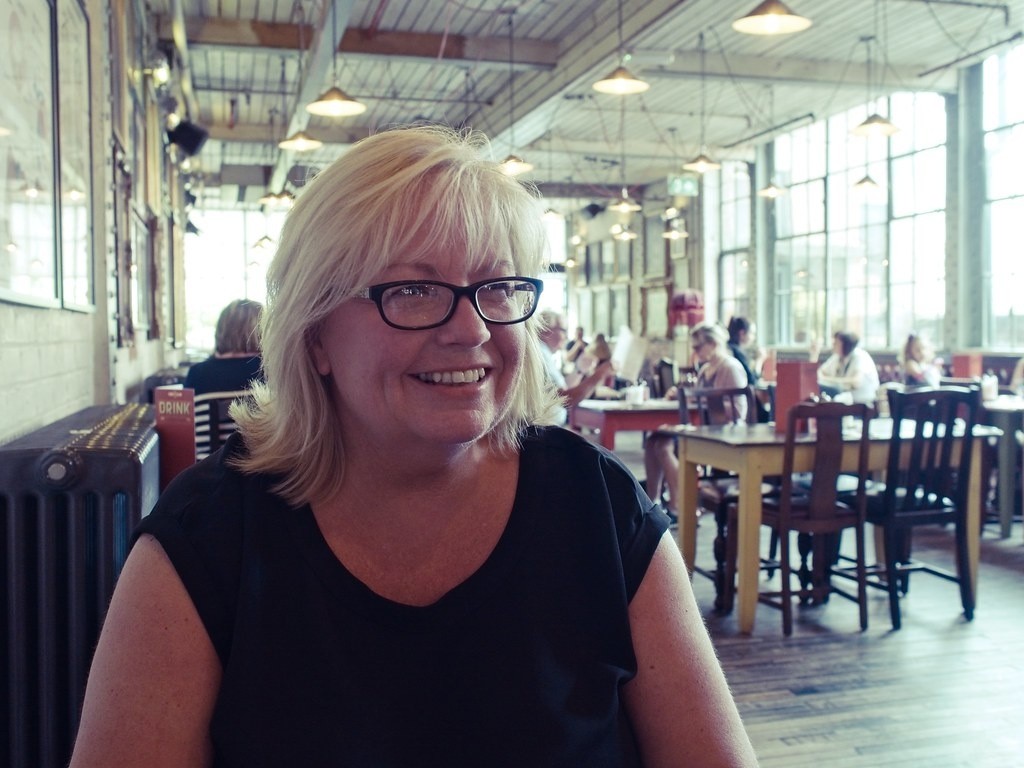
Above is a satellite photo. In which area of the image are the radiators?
[0,401,159,768]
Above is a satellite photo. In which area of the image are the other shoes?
[665,508,679,528]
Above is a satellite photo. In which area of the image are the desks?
[657,417,1004,631]
[568,396,707,453]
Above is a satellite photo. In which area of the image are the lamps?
[253,0,897,259]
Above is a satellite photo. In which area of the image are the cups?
[627,387,643,406]
[682,372,695,393]
[981,375,998,400]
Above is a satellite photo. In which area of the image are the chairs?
[647,359,981,639]
[210,395,256,454]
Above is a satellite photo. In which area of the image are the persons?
[817,331,880,403]
[902,332,943,386]
[726,316,768,386]
[69,125,760,768]
[536,310,612,426]
[584,331,610,362]
[644,322,748,526]
[183,299,264,454]
[566,326,588,362]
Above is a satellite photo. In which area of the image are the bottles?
[800,391,819,433]
[817,391,831,403]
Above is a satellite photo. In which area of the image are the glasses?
[692,342,707,353]
[323,277,543,330]
